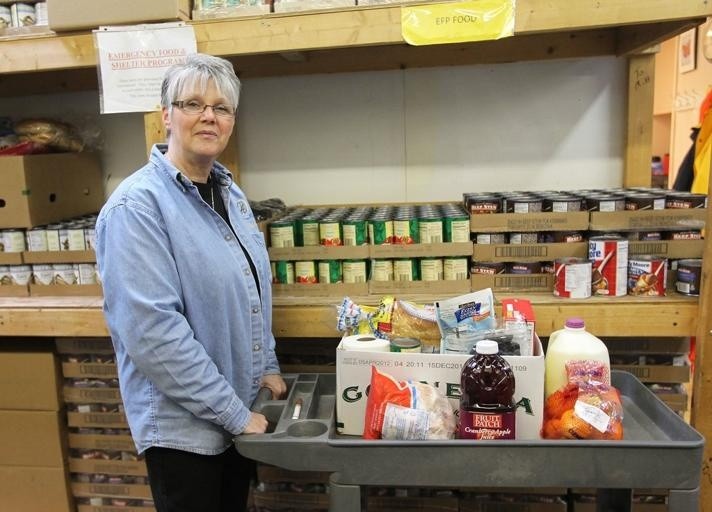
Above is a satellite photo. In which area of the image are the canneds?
[0,188,706,299]
[390,337,422,353]
[0,2,48,28]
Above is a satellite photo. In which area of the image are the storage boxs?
[335,330,544,440]
[0,152,105,229]
[0,352,73,512]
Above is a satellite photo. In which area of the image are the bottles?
[652,156,665,176]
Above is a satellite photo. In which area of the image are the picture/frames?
[676,26,697,74]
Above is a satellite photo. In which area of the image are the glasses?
[170,100,238,118]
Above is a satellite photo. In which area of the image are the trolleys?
[232,369,706,512]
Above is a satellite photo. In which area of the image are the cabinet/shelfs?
[0,0,712,512]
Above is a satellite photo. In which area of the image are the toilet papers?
[342,334,390,354]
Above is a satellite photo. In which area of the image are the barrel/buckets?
[544,318,611,402]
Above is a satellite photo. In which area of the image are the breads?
[14,119,57,135]
[20,133,84,151]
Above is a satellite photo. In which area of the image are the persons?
[93,53,287,511]
